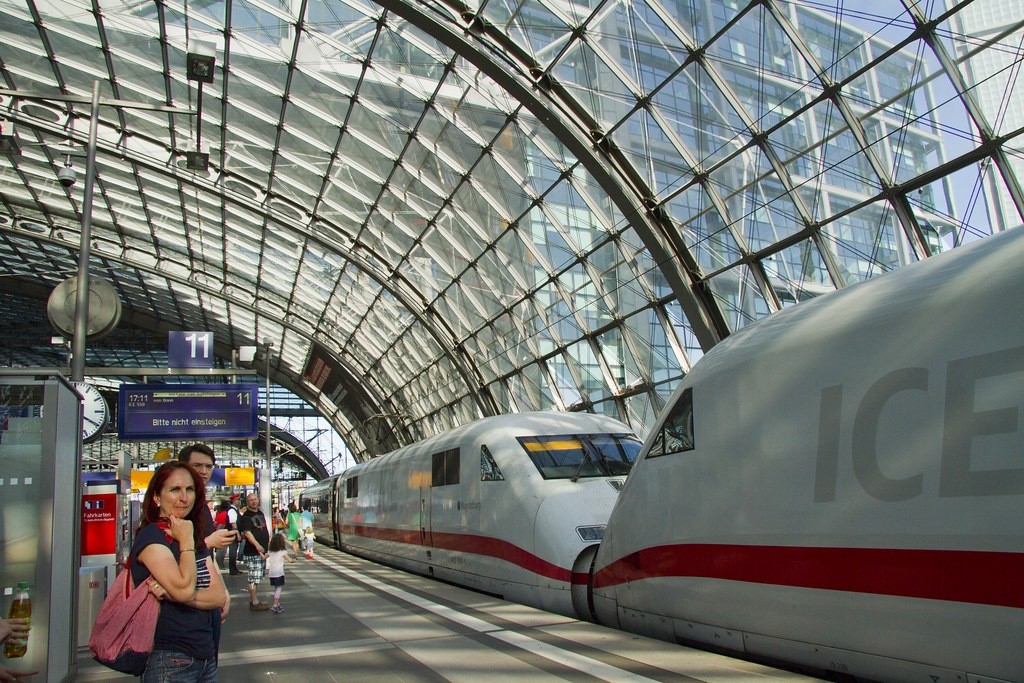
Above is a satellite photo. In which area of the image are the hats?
[229,494,240,500]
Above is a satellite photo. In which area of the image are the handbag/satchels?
[89,517,173,676]
[277,519,284,528]
[298,528,305,541]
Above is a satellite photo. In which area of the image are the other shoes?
[306,554,313,559]
[230,571,243,575]
[294,554,298,559]
[250,601,269,611]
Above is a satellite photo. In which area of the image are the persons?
[127,460,226,683]
[177,442,238,671]
[211,493,318,616]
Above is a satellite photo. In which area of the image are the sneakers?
[271,603,284,613]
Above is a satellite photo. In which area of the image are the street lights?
[262,336,274,557]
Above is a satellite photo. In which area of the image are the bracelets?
[179,549,195,552]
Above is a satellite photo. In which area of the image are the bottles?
[4,581,31,658]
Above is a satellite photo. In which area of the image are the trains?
[590,222,1024,683]
[299,411,645,624]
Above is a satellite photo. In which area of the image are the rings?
[155,583,159,588]
